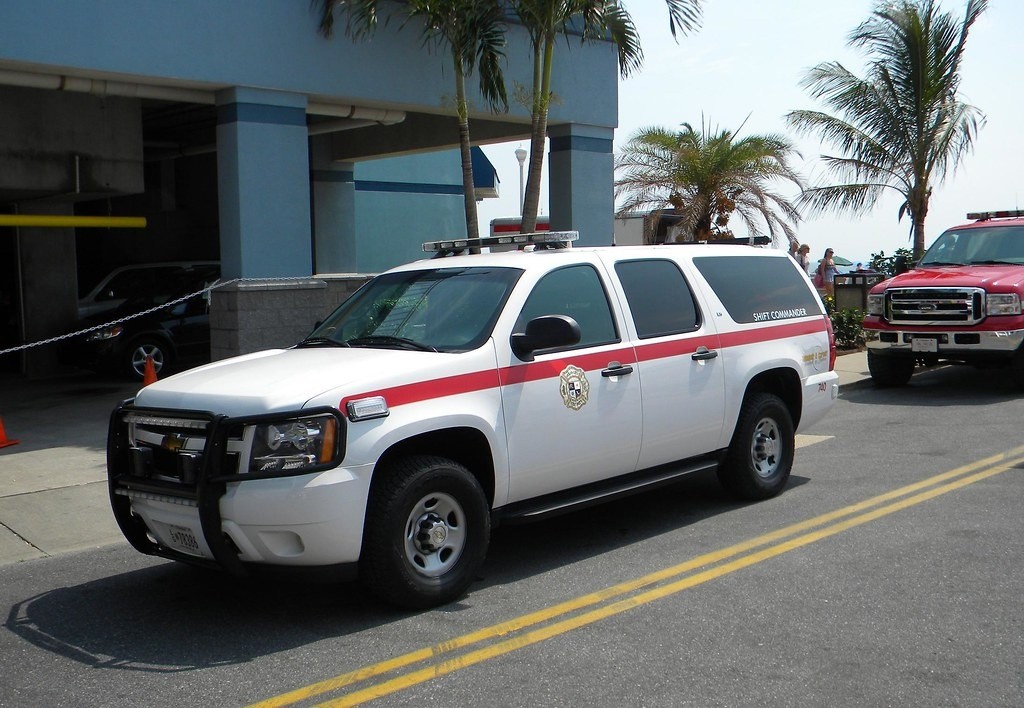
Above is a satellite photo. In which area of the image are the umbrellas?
[818,256,853,266]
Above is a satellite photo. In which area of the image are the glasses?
[830,252,833,253]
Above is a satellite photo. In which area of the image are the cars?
[63,259,224,380]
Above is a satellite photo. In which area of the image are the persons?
[821,248,846,297]
[936,236,956,262]
[856,254,912,281]
[789,242,809,271]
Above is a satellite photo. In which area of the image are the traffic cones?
[142,355,159,387]
[0,417,19,447]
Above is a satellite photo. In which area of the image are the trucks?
[488,205,724,253]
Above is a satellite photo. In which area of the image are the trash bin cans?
[831,270,885,316]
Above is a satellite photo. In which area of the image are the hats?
[826,248,834,252]
[800,244,809,251]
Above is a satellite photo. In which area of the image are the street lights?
[514,141,528,216]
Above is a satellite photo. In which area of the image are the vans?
[104,229,844,610]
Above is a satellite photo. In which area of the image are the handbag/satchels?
[814,259,827,288]
[800,255,805,269]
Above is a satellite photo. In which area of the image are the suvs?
[861,210,1024,388]
